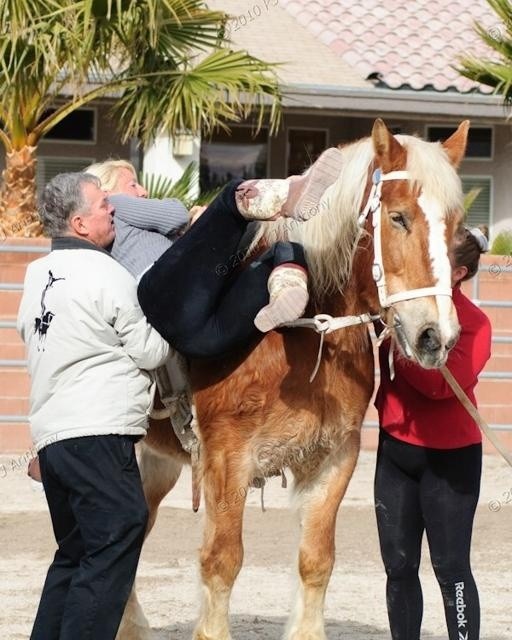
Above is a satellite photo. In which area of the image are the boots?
[234,147,343,221]
[253,263,310,334]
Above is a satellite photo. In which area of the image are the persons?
[15,173,170,640]
[82,147,344,359]
[375,227,493,640]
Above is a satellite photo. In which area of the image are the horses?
[113,115,472,639]
